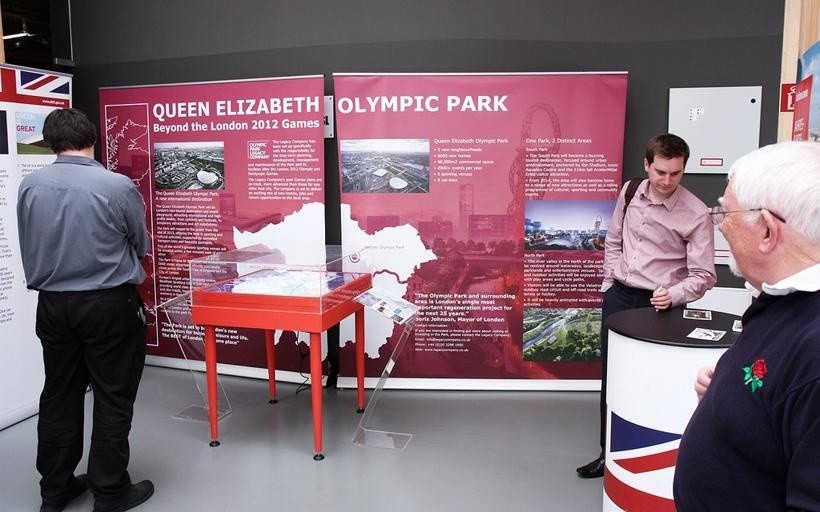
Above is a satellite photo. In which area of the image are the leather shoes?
[40,474,90,512]
[93,480,154,512]
[576,457,605,478]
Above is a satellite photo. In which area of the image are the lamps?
[3,12,50,40]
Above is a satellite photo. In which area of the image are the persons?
[666,138,819,511]
[15,108,155,511]
[573,131,717,476]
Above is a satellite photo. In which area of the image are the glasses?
[709,206,786,225]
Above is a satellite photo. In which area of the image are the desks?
[601,304,743,512]
